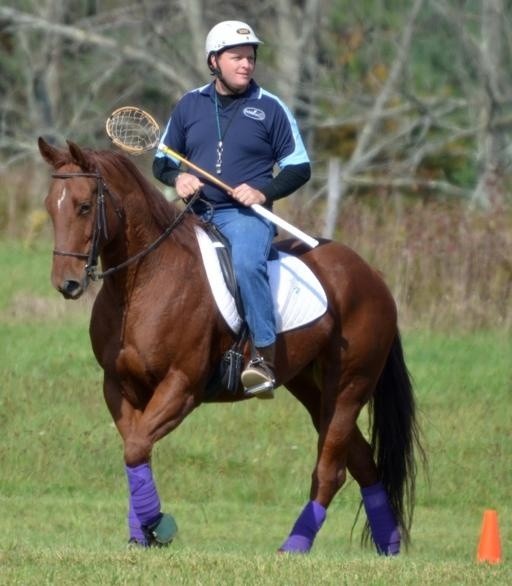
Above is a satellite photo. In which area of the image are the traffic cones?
[479,508,502,575]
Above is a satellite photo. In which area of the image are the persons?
[151,19,311,401]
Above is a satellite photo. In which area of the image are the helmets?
[203,18,264,67]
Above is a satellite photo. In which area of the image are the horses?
[37,136,431,557]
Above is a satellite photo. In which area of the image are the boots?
[242,345,276,398]
